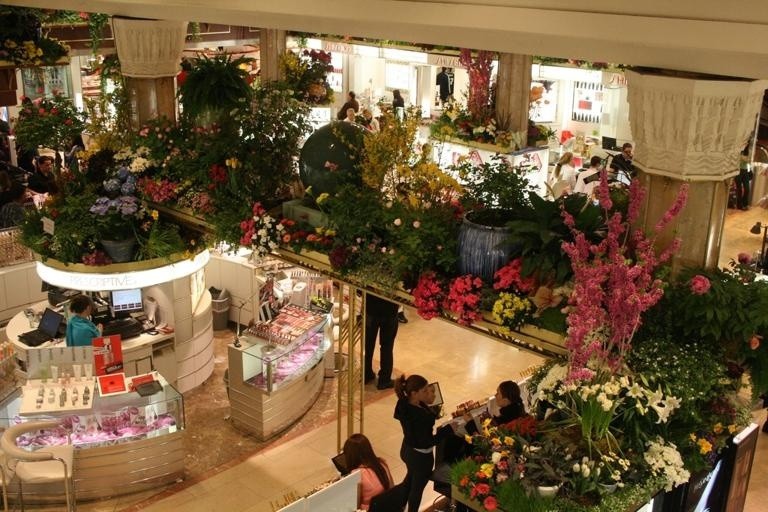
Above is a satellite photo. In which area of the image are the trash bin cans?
[208,286,232,330]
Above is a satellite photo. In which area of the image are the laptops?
[17,308,63,346]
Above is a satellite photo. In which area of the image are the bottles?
[35,386,90,409]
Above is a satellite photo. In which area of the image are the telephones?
[135,314,155,333]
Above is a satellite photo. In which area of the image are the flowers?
[2,3,557,291]
[413,166,766,511]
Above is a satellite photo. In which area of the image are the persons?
[63,295,104,347]
[0,136,85,229]
[547,143,635,202]
[736,139,750,211]
[343,433,394,511]
[462,379,528,459]
[436,67,455,105]
[425,384,437,405]
[356,288,396,390]
[337,87,407,132]
[364,375,446,511]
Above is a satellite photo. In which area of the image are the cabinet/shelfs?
[1,248,362,504]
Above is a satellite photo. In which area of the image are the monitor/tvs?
[110,288,143,313]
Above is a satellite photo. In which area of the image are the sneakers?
[397,311,408,323]
[365,372,375,385]
[377,379,395,390]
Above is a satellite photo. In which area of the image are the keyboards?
[102,323,144,339]
